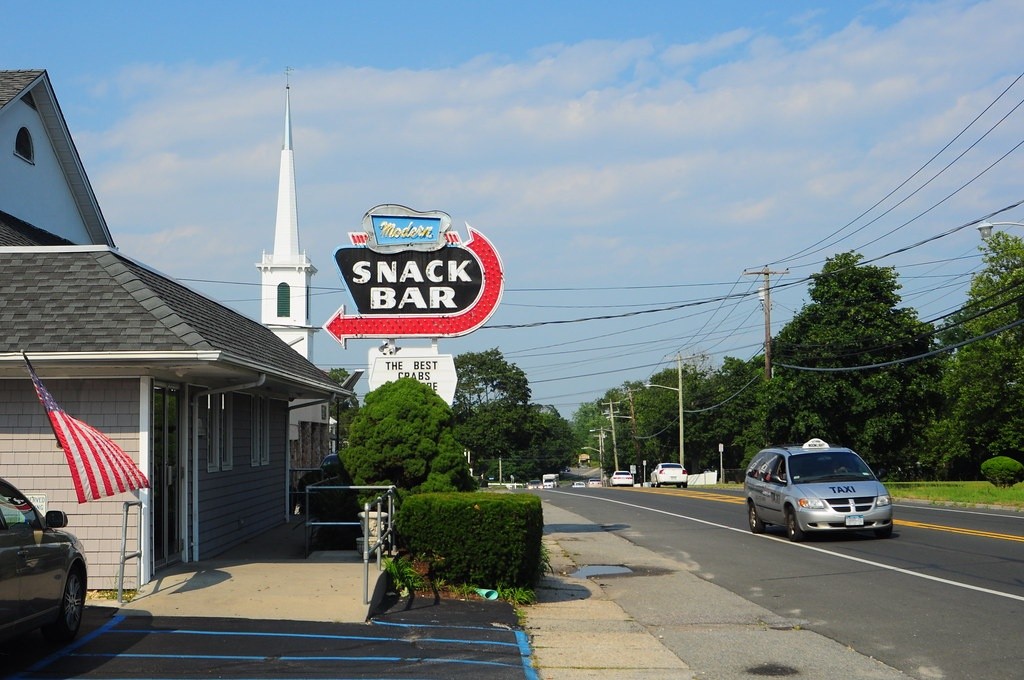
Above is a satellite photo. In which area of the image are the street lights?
[589,428,619,470]
[581,445,607,487]
[644,384,685,468]
[605,415,644,487]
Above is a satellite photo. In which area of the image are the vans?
[742,445,893,542]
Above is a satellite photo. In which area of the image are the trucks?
[543,473,560,489]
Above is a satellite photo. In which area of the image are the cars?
[528,480,543,491]
[570,480,585,487]
[650,463,688,489]
[0,478,87,642]
[610,470,634,487]
[587,479,603,488]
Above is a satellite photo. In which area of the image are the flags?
[20,350,150,504]
